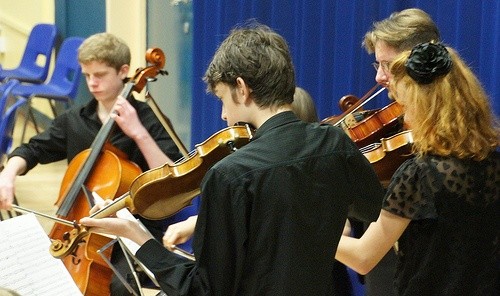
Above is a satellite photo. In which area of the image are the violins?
[359,129,417,180]
[49,126,255,259]
[349,102,407,148]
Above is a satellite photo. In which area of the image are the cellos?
[318,95,362,127]
[48,48,169,296]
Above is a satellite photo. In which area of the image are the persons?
[360,8,500,296]
[335,41,500,296]
[292,87,323,125]
[79,23,384,296]
[0,32,184,296]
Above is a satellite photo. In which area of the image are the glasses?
[372,60,396,72]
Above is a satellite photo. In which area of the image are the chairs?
[0,24,57,136]
[0,78,17,119]
[0,97,29,220]
[12,37,86,145]
